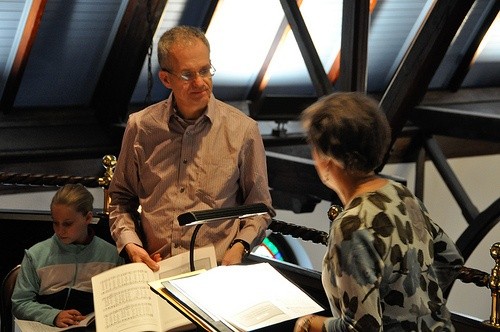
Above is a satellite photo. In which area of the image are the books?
[13,311,95,332]
[147,262,325,332]
[91,243,217,332]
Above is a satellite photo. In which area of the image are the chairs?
[1,264,35,332]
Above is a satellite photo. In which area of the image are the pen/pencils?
[73,314,76,319]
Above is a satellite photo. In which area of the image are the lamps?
[177,202,269,271]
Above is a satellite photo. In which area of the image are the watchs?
[229,239,251,260]
[302,315,316,332]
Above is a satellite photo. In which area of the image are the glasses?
[161,63,215,81]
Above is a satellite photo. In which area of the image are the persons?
[107,26,276,272]
[294,92,465,332]
[11,183,125,332]
